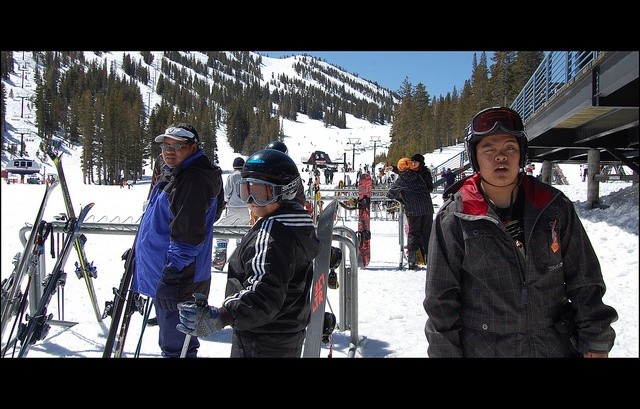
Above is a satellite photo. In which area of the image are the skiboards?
[102,212,139,359]
[1,176,95,359]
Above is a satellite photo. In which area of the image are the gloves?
[176,301,224,337]
[155,276,185,313]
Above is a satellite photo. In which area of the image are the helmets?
[397,158,413,173]
[240,149,300,205]
[265,141,288,156]
[465,105,528,187]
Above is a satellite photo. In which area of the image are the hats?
[411,154,425,165]
[154,122,199,144]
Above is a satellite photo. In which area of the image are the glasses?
[466,106,524,142]
[158,143,194,151]
[235,170,299,207]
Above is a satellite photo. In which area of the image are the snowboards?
[303,197,340,359]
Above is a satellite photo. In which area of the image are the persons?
[422,106,619,357]
[411,153,433,195]
[133,121,226,357]
[176,149,322,358]
[442,169,455,188]
[301,156,398,188]
[387,157,434,268]
[210,157,250,272]
